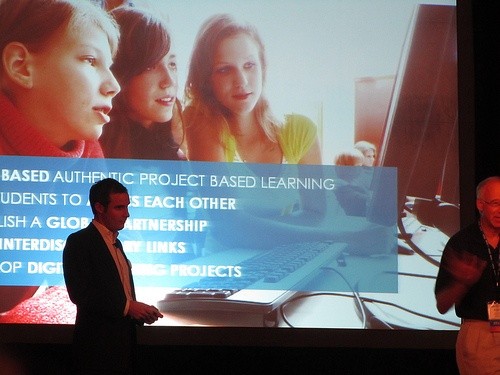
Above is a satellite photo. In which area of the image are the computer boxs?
[354,75,397,148]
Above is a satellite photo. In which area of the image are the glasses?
[481,199,500,207]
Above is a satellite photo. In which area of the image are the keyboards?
[159,241,348,316]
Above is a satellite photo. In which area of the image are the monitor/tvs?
[366,3,457,225]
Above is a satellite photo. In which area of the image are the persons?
[434,176,500,375]
[63,178,163,375]
[0,0,396,314]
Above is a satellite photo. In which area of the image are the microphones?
[115,243,120,247]
